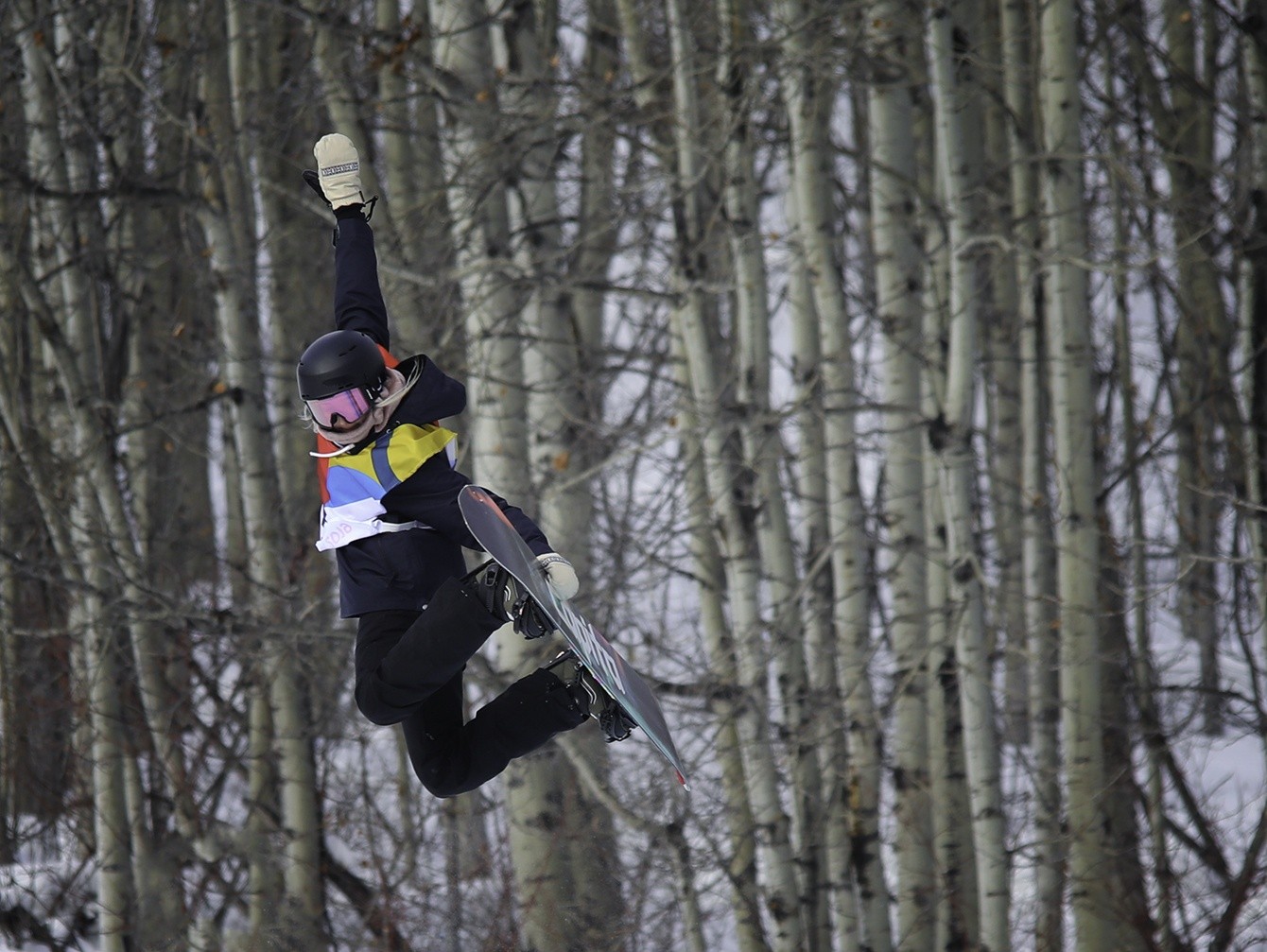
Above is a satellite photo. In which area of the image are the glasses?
[303,384,373,430]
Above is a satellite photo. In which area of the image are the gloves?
[532,552,579,599]
[301,133,367,216]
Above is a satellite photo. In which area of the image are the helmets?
[296,330,387,411]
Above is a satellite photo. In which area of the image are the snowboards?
[456,483,691,793]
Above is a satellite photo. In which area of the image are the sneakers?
[544,650,634,741]
[474,563,547,639]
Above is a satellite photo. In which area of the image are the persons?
[296,132,640,798]
